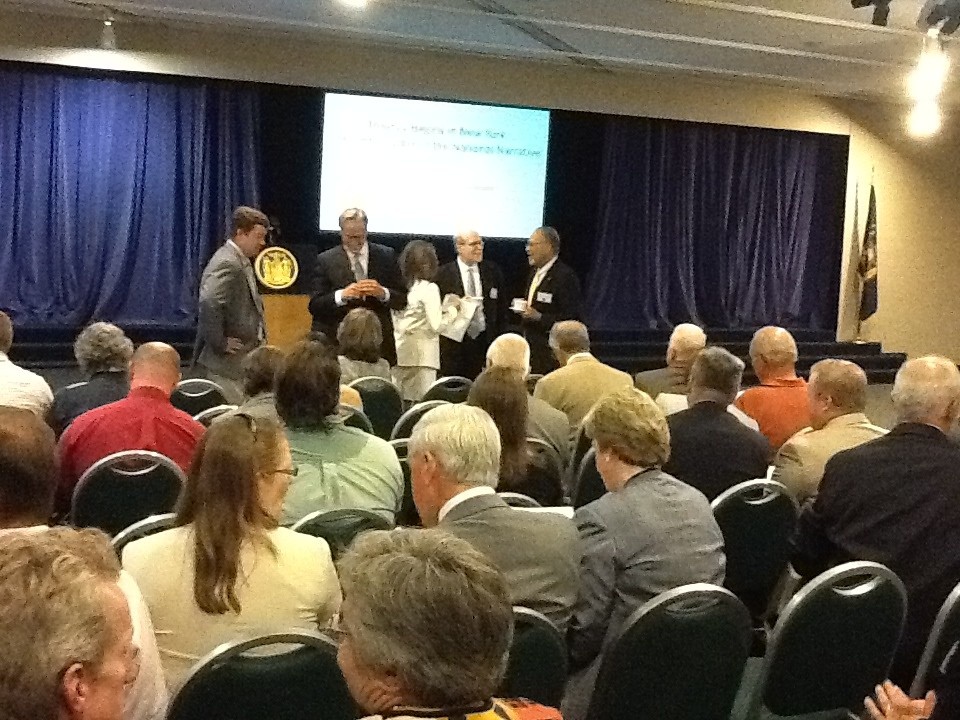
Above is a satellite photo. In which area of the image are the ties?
[467,267,480,339]
[353,252,365,283]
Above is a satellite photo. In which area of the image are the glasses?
[469,242,485,246]
[265,465,301,479]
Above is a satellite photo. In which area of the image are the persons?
[0,525,138,720]
[390,240,461,413]
[560,385,726,720]
[308,208,407,368]
[0,307,960,720]
[855,677,960,720]
[430,229,509,388]
[408,404,582,639]
[121,405,343,698]
[337,528,564,720]
[521,227,579,376]
[182,206,270,379]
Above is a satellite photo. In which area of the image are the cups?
[509,298,527,314]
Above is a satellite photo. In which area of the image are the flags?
[855,185,877,321]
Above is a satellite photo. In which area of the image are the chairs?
[54,377,960,720]
